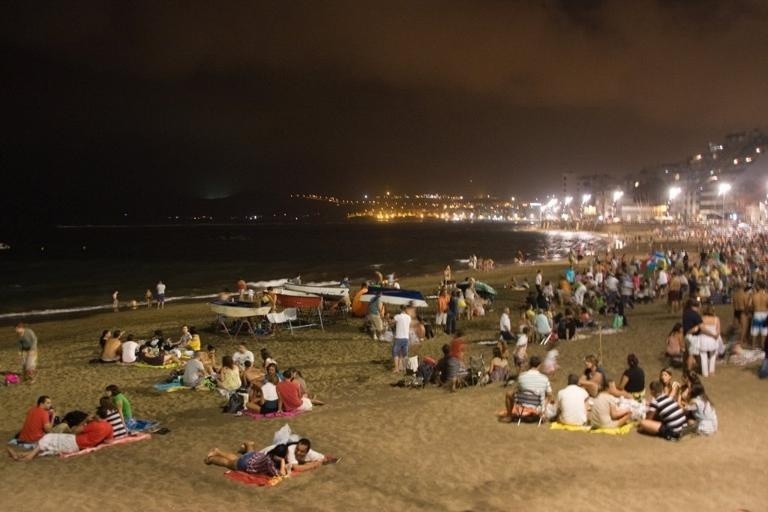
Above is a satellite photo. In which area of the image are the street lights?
[717,183,732,224]
[539,190,626,224]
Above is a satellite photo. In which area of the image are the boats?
[205,274,428,318]
[455,279,498,296]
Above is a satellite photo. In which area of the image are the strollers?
[467,350,488,389]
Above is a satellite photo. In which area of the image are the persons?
[9,384,134,461]
[205,438,337,479]
[366,199,767,388]
[218,279,277,312]
[97,324,324,414]
[14,322,38,385]
[113,280,166,313]
[500,355,717,440]
[340,277,351,288]
[291,273,302,285]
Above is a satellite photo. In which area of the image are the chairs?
[456,352,488,391]
[514,388,548,427]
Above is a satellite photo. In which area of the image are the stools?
[538,333,555,347]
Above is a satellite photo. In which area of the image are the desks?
[205,284,441,345]
